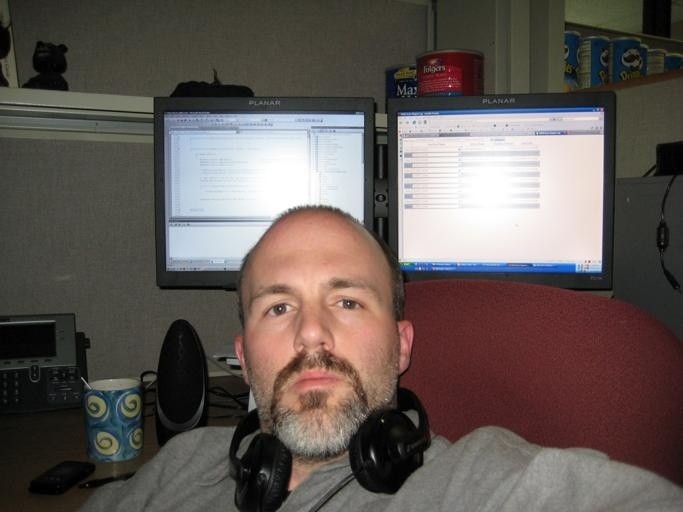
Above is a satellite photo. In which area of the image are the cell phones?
[28,460,95,496]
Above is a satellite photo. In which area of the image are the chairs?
[399,278,681,489]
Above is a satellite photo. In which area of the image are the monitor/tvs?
[387,91,617,292]
[153,96,375,289]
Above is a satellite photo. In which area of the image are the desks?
[1,375,252,512]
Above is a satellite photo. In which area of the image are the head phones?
[229,388,431,512]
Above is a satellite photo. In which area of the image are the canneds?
[563,30,683,93]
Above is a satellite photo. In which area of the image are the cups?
[83,378,146,463]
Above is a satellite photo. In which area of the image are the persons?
[77,204,680,510]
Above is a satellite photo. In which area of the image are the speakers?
[154,318,208,446]
